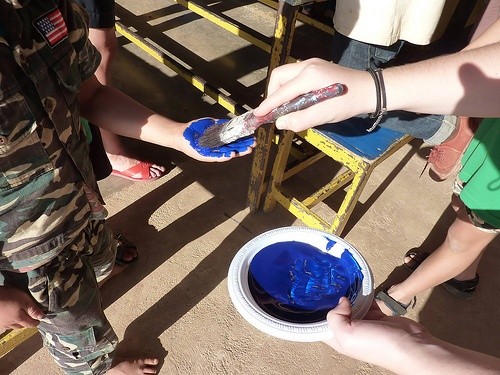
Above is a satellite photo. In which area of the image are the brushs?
[198,84,344,149]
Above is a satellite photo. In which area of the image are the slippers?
[110,160,168,183]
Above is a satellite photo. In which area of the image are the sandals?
[114,234,138,266]
[364,290,408,322]
[403,251,482,298]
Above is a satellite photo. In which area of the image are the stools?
[261,107,415,239]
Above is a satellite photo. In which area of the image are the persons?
[332,0,479,181]
[0,0,256,375]
[82,0,166,182]
[253,21,500,375]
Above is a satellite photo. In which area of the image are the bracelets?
[364,67,387,132]
